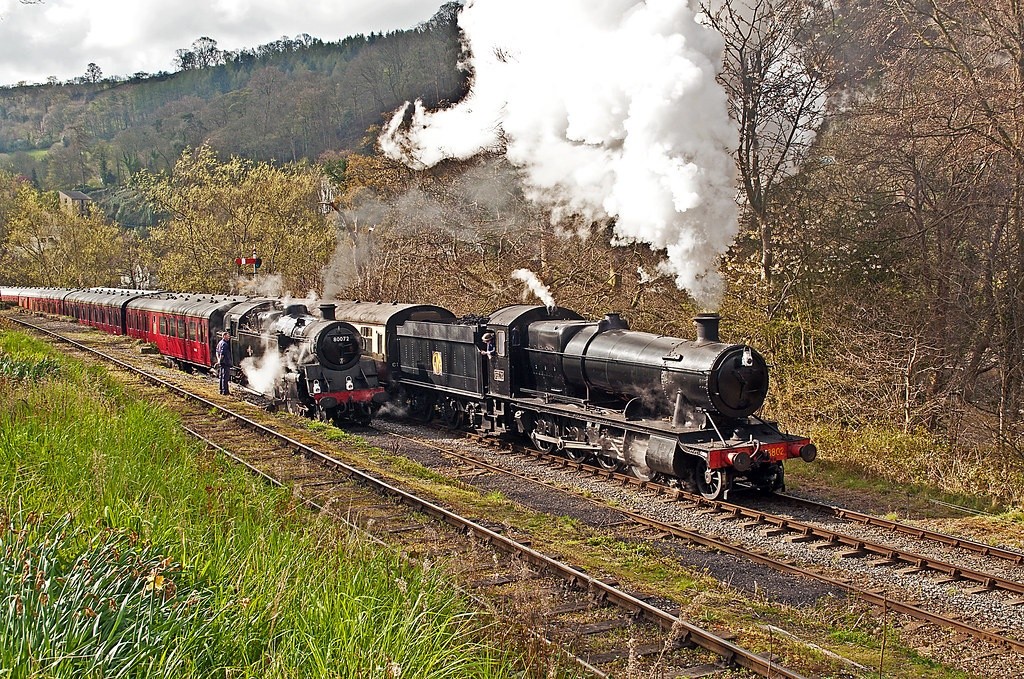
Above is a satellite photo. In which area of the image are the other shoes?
[221,392,234,396]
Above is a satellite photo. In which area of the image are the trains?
[0,285,818,503]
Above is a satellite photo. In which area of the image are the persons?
[215,331,233,394]
